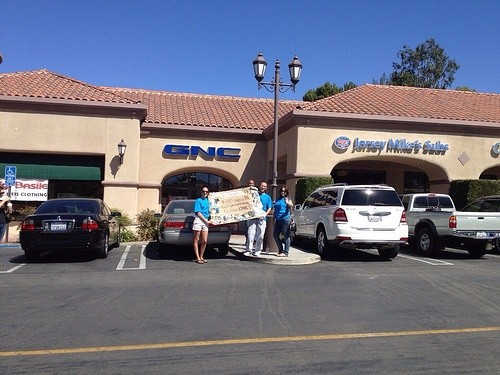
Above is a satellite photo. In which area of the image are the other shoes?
[197,258,208,264]
[275,251,288,257]
[254,250,263,256]
[243,251,253,256]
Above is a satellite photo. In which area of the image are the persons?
[243,180,272,257]
[271,185,293,257]
[0,179,9,241]
[192,187,213,264]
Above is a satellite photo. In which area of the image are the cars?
[458,195,500,254]
[154,199,230,256]
[19,198,121,258]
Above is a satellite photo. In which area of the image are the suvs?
[290,183,409,258]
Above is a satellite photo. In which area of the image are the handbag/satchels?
[289,218,297,233]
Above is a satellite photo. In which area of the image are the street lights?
[252,52,303,253]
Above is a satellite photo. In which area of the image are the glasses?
[202,190,209,193]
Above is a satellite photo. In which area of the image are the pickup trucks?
[399,193,500,258]
